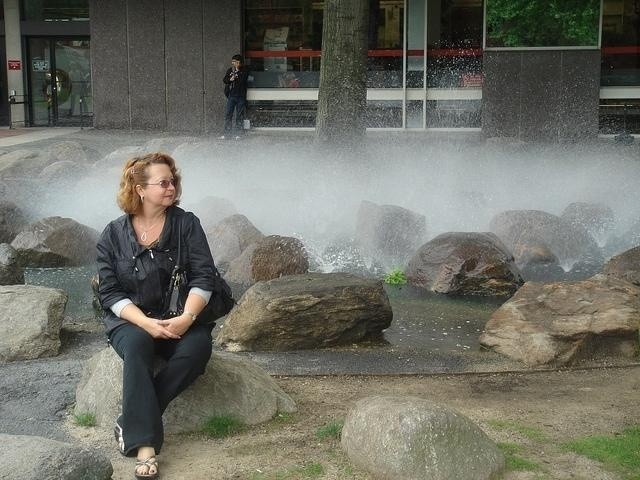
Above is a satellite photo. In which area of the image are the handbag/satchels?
[159,223,237,326]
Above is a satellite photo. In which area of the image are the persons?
[88,150,215,480]
[214,53,249,142]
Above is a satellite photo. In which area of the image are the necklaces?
[135,215,163,242]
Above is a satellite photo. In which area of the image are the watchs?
[183,313,197,321]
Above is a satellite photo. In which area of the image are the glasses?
[148,180,177,188]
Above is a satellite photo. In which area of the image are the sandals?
[114,421,126,456]
[133,453,160,479]
